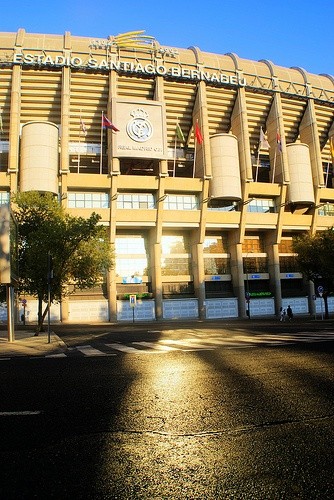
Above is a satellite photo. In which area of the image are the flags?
[103,114,119,132]
[260,130,271,151]
[276,133,283,152]
[330,139,334,163]
[195,122,203,146]
[176,123,186,143]
[80,115,88,136]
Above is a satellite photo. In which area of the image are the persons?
[280,306,294,322]
